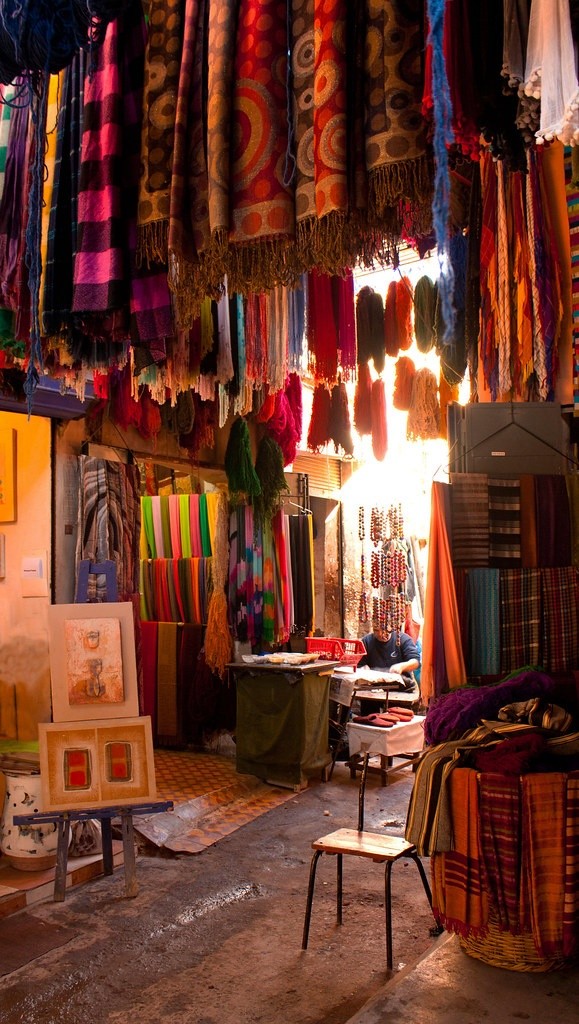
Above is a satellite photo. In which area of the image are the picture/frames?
[37,715,159,814]
[46,601,141,724]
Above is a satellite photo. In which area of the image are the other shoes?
[352,706,415,727]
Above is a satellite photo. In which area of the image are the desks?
[225,660,342,793]
[329,665,401,786]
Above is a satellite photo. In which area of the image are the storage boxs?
[305,636,368,667]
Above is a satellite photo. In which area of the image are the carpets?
[0,912,80,979]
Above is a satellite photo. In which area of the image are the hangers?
[439,400,579,478]
[78,403,140,466]
[280,493,315,517]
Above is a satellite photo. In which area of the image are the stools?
[348,715,426,787]
[300,825,443,973]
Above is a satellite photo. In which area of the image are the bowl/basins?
[242,652,320,666]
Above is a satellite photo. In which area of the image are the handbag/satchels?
[67,813,102,856]
[404,604,421,644]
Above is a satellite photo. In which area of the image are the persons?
[357,612,421,682]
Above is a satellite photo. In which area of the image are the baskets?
[436,769,579,973]
[0,824,73,871]
[305,635,368,672]
